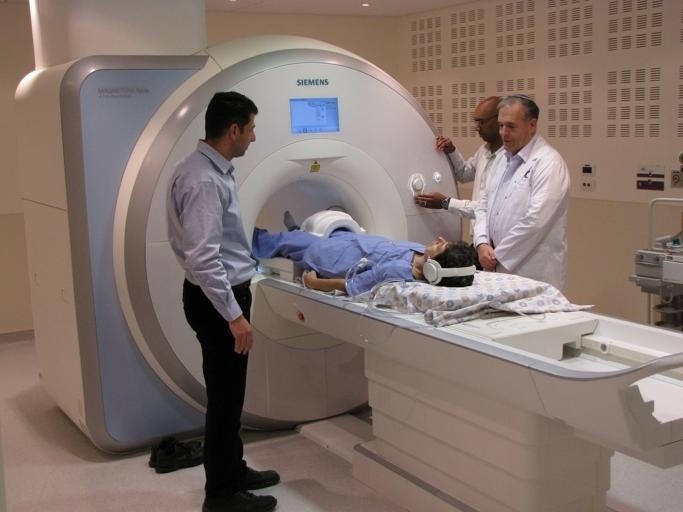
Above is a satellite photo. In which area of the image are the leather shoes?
[148,436,205,473]
[202,467,280,512]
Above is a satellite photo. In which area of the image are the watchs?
[441,196,450,210]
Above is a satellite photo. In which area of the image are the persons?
[414,96,503,245]
[165,90,281,512]
[472,93,570,296]
[251,210,477,297]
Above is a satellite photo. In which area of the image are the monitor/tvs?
[288,96,339,134]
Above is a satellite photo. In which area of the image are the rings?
[419,201,427,206]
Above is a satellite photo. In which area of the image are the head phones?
[421,257,478,286]
